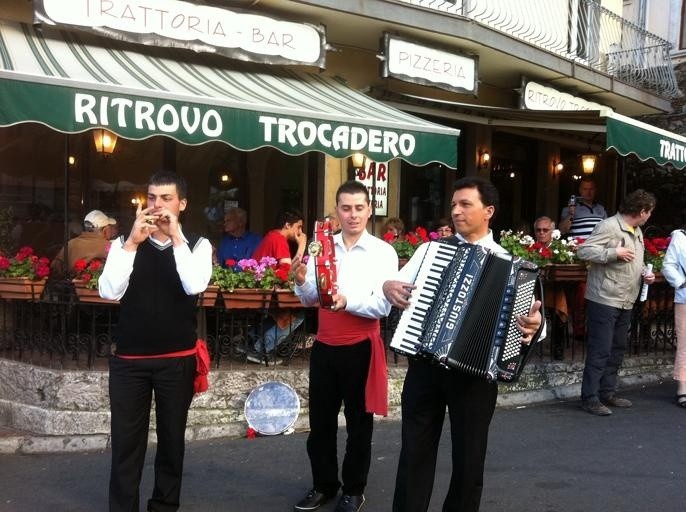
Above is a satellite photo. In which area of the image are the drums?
[308,220,337,308]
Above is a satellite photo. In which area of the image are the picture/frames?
[351,155,390,218]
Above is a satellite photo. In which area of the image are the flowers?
[644,237,672,273]
[384,227,440,260]
[551,229,584,265]
[0,246,51,281]
[211,255,296,289]
[75,259,105,290]
[500,229,551,267]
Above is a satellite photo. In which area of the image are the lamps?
[93,128,117,159]
[552,158,565,179]
[479,151,490,169]
[578,143,597,174]
[348,153,363,176]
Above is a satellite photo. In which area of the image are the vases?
[536,263,587,283]
[652,272,663,283]
[1,276,49,301]
[398,258,408,266]
[220,286,275,311]
[275,285,320,308]
[195,285,218,308]
[72,277,121,305]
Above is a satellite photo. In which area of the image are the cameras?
[570,194,584,205]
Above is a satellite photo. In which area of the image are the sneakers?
[235,341,283,366]
[600,391,633,409]
[579,400,613,417]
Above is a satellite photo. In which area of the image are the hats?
[82,209,117,229]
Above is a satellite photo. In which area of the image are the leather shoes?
[328,494,367,512]
[292,488,328,509]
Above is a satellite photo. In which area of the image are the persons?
[247,208,307,365]
[291,180,399,512]
[50,210,117,274]
[661,227,686,408]
[434,217,455,239]
[558,176,607,240]
[379,176,547,512]
[212,207,263,274]
[386,218,404,238]
[577,189,656,414]
[529,217,565,361]
[98,171,213,512]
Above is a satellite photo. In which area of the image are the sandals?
[672,391,685,408]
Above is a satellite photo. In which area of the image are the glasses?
[534,227,553,233]
[434,228,452,233]
